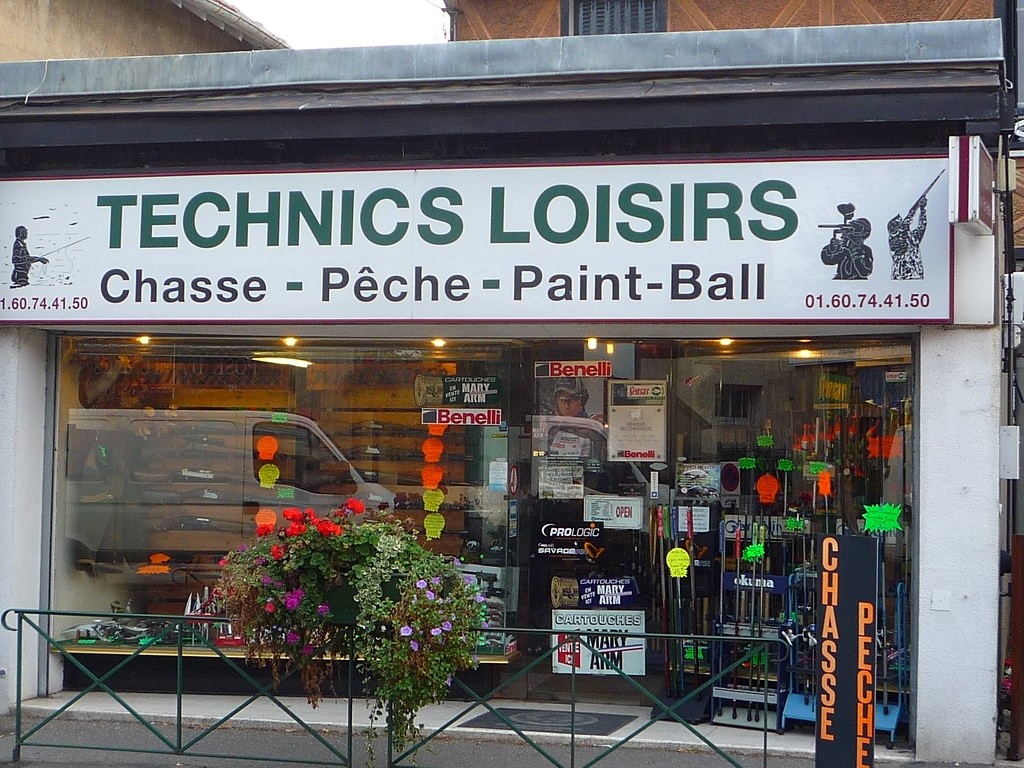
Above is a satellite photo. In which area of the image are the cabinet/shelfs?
[337,428,475,535]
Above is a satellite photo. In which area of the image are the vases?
[318,570,456,626]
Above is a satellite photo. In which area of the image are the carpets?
[456,708,639,736]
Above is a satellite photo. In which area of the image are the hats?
[553,377,589,405]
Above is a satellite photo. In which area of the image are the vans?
[60,410,396,561]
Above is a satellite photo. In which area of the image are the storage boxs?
[460,563,518,656]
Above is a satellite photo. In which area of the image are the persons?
[541,378,605,424]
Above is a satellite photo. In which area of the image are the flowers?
[209,498,490,768]
